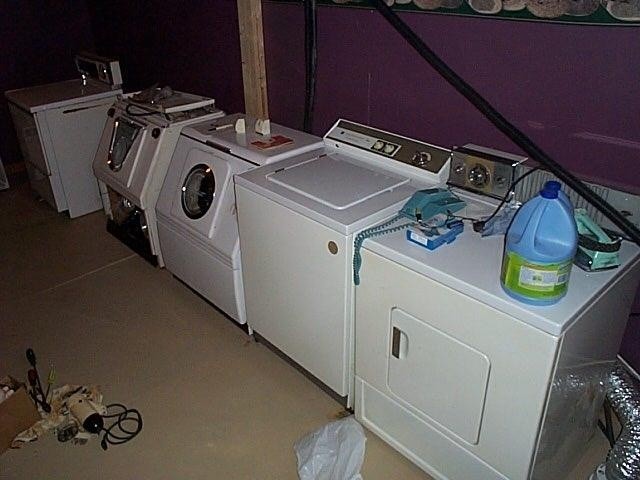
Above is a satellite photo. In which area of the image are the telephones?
[398,189,466,219]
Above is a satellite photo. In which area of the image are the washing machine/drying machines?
[355,145,638,480]
[233,120,451,399]
[155,111,321,325]
[92,85,225,270]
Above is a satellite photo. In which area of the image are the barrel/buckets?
[499,180,578,305]
